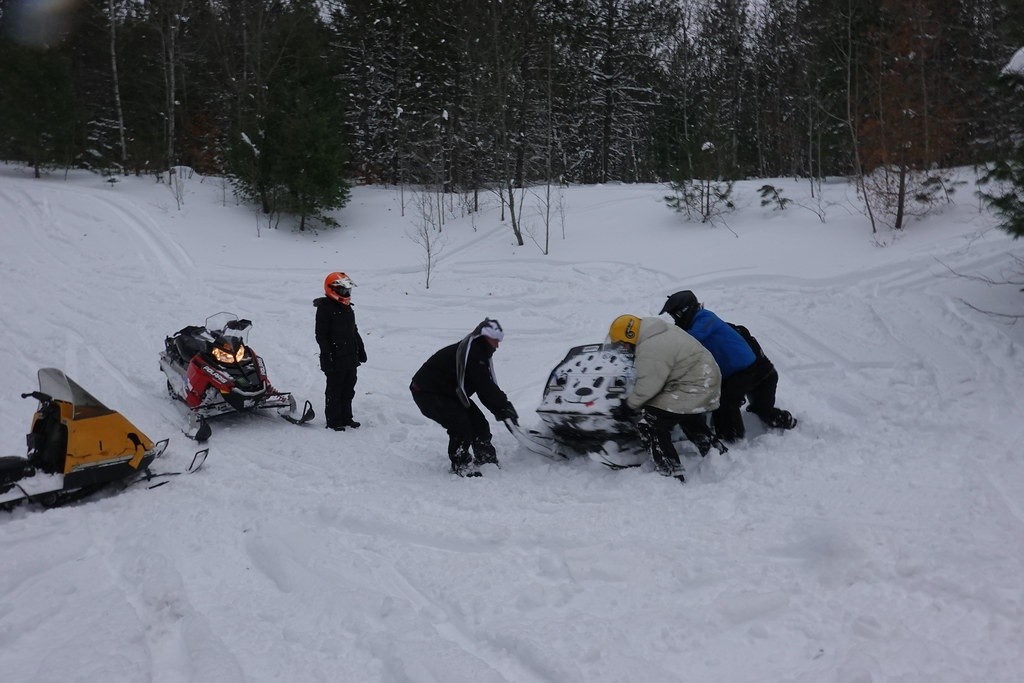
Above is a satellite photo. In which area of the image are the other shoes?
[674,473,685,482]
[475,455,498,467]
[770,410,798,430]
[452,461,482,477]
[343,419,361,428]
[325,424,345,432]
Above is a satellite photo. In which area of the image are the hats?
[473,319,504,342]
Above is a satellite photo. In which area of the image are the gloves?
[495,401,520,426]
[359,349,367,363]
[320,352,335,371]
[609,397,636,422]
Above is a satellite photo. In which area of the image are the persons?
[407,317,521,479]
[656,289,757,445]
[724,321,800,430]
[606,314,722,483]
[312,272,369,432]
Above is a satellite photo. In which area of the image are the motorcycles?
[160,311,317,444]
[0,366,211,519]
[500,330,714,471]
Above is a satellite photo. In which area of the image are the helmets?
[323,271,351,306]
[658,290,702,328]
[609,314,641,345]
